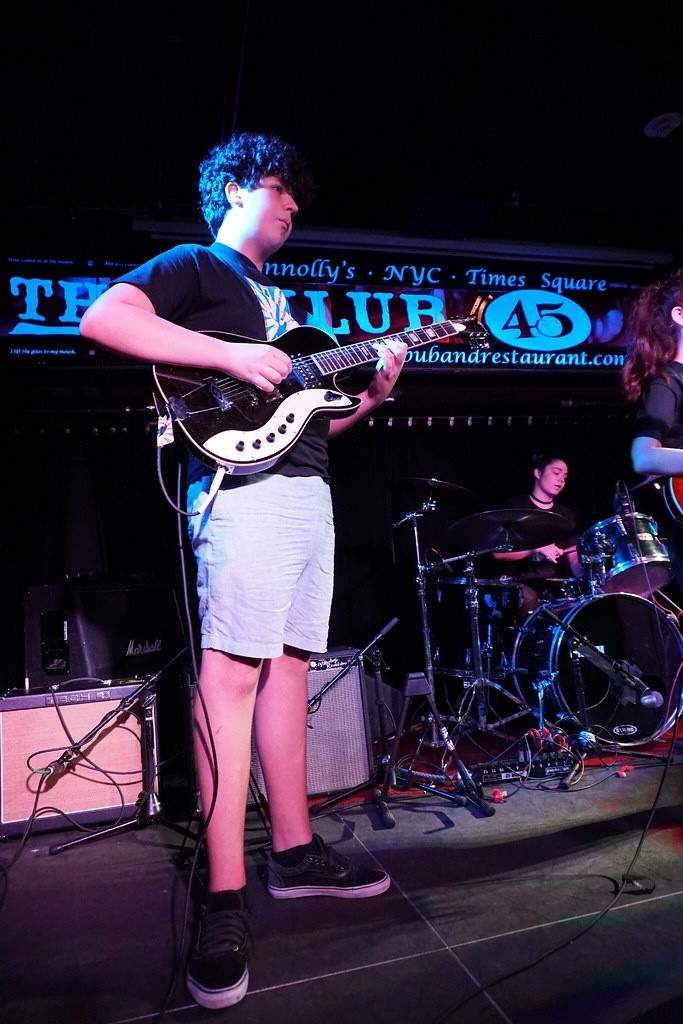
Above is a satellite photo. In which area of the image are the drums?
[423,576,522,681]
[576,512,676,600]
[514,591,683,742]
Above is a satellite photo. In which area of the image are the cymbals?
[443,507,579,553]
[377,475,480,510]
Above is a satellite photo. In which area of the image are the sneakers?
[186,890,252,1009]
[268,833,391,899]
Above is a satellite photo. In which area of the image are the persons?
[81,136,408,1010]
[492,452,583,617]
[621,270,682,585]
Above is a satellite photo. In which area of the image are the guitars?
[147,294,493,475]
[661,472,683,526]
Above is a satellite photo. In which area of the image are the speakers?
[181,645,377,818]
[68,580,191,679]
[363,669,404,740]
[0,683,161,839]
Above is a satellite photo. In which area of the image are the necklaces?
[530,493,554,504]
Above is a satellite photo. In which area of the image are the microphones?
[613,481,621,515]
[642,691,664,708]
[45,762,65,776]
[432,548,454,575]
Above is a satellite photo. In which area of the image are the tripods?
[308,488,673,813]
[48,647,207,855]
